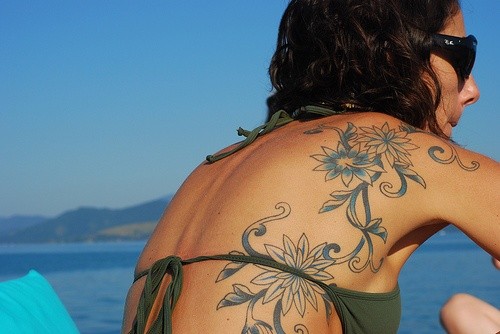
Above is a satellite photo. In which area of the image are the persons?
[121,0,500,334]
[438,258,500,334]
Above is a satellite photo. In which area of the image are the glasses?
[430,34,478,93]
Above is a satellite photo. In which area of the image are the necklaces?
[338,103,364,108]
